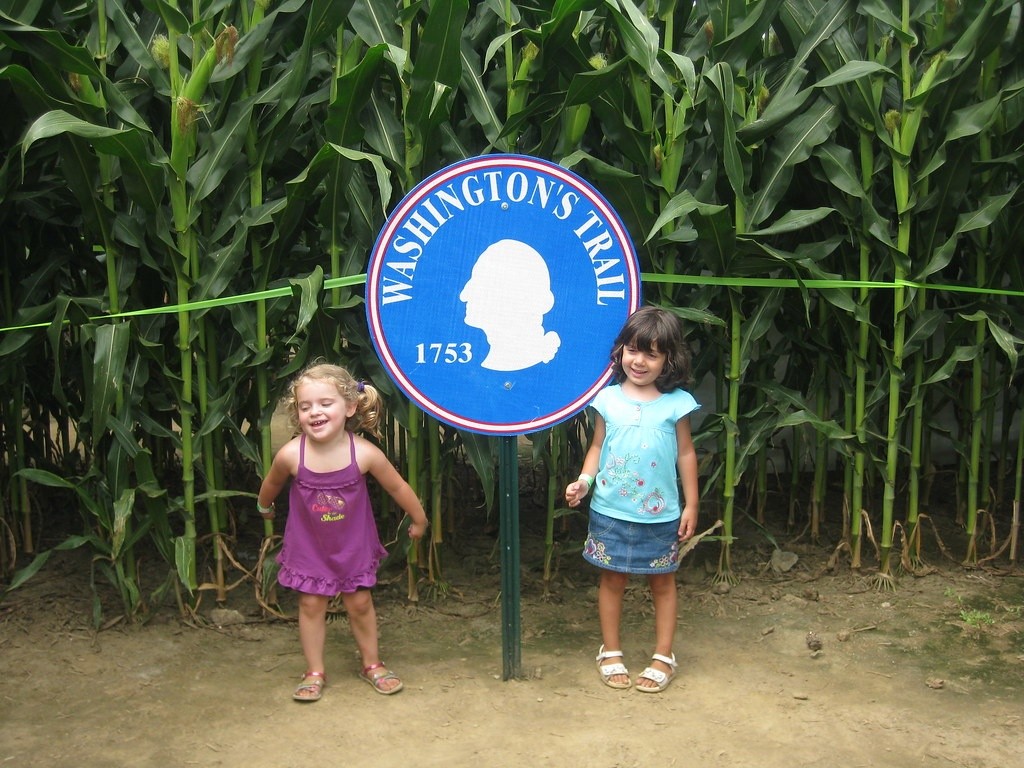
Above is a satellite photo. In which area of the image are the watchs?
[257,502,276,513]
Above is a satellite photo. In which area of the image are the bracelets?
[578,474,594,490]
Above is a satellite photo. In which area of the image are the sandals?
[358,661,403,694]
[595,644,632,689]
[636,653,679,693]
[293,672,327,700]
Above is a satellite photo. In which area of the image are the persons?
[565,305,702,694]
[256,363,429,702]
